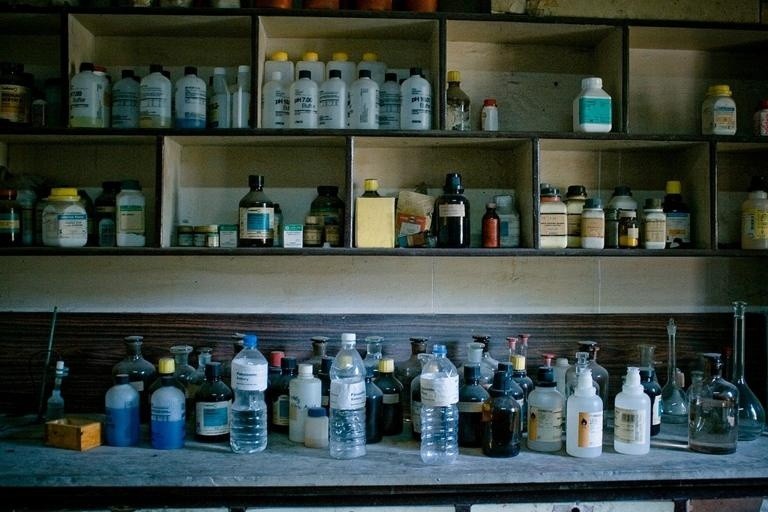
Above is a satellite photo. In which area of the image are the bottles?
[444,68,612,135]
[353,171,519,249]
[0,62,60,137]
[741,190,767,250]
[538,182,691,249]
[699,83,738,137]
[43,360,66,421]
[1,175,147,249]
[69,58,248,127]
[174,175,344,248]
[101,332,363,461]
[362,299,765,463]
[261,49,435,134]
[753,100,768,143]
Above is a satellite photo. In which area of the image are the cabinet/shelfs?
[0,0,768,256]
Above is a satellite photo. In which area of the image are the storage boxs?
[42,415,105,454]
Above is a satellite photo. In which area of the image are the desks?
[0,412,765,511]
